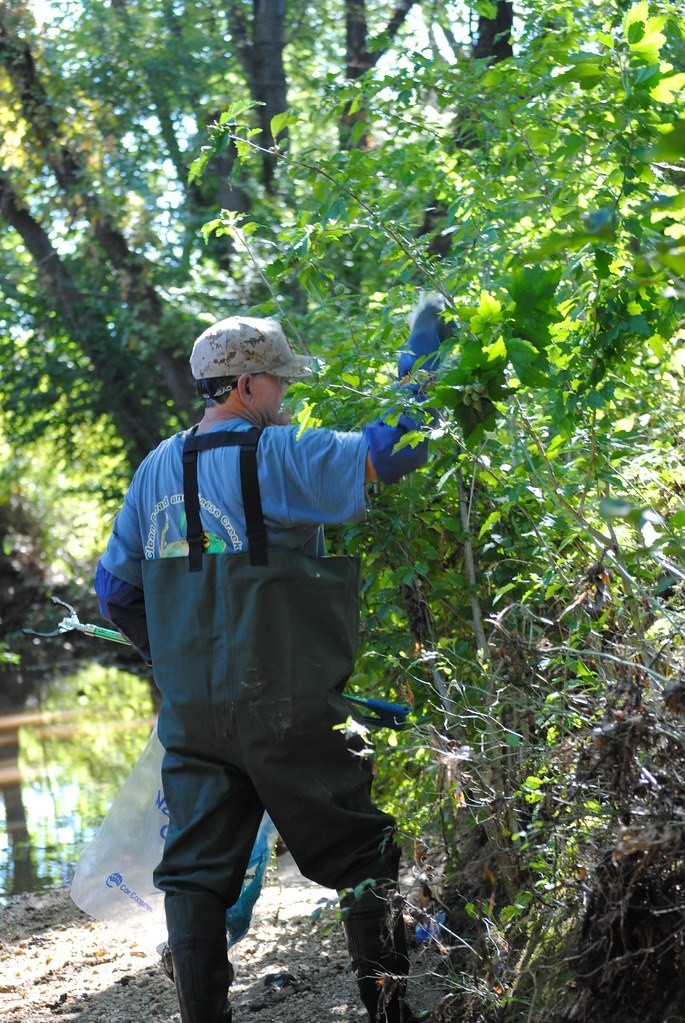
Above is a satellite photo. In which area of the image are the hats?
[188,315,324,380]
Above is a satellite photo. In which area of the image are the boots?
[343,903,437,1023]
[162,944,234,1023]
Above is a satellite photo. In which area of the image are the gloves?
[362,295,460,486]
[93,561,150,662]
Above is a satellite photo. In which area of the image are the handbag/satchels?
[68,717,270,958]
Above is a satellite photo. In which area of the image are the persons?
[94,296,456,1023]
